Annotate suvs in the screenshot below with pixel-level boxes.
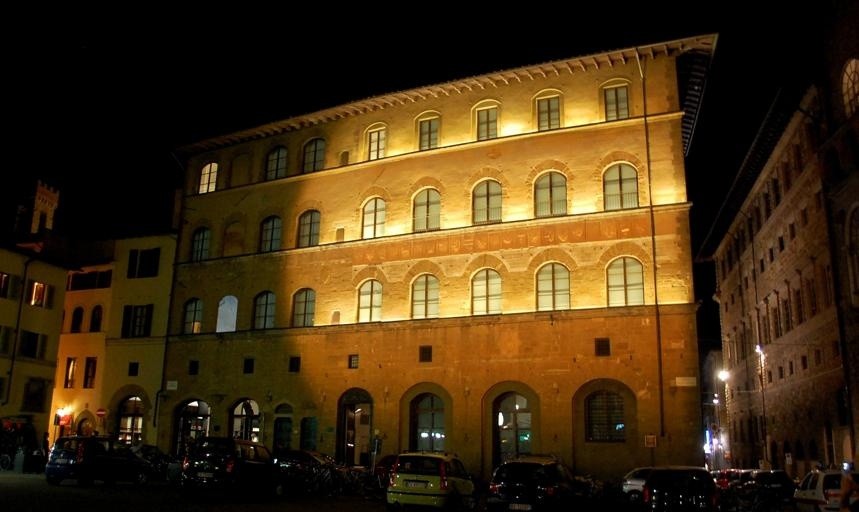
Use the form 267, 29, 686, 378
618, 465, 858, 512
386, 449, 605, 512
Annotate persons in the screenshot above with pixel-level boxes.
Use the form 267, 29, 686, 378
0, 415, 39, 474
39, 432, 51, 472
233, 431, 240, 440
91, 430, 98, 435
272, 439, 288, 458
250, 433, 258, 449
838, 459, 855, 512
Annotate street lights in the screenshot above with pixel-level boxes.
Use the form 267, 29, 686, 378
753, 344, 770, 462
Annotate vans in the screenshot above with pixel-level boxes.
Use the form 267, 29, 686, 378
179, 436, 271, 494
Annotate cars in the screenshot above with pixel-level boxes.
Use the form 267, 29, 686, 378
271, 448, 370, 499
45, 435, 180, 488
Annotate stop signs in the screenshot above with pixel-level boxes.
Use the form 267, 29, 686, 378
96, 407, 108, 419
